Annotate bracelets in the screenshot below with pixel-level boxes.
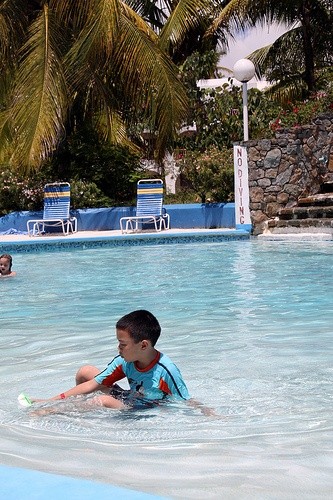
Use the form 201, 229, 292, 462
60, 393, 65, 400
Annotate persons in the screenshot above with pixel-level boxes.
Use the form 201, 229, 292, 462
0, 254, 17, 276
26, 309, 213, 420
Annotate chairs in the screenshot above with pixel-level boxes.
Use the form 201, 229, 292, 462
119, 179, 170, 234
27, 181, 78, 236
